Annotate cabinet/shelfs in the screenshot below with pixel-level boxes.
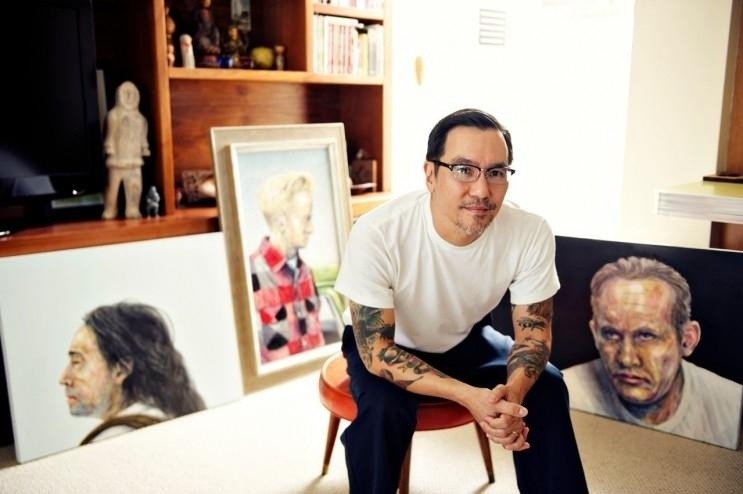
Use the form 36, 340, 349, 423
0, 0, 393, 258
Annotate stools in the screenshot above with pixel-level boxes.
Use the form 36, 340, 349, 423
318, 351, 504, 494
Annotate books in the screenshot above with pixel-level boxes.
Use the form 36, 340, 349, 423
313, 14, 384, 79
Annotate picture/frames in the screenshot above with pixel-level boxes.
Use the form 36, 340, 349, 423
206, 121, 365, 396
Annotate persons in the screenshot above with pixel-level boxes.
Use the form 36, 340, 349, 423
249, 176, 326, 365
60, 302, 207, 445
334, 108, 589, 494
146, 185, 160, 218
559, 256, 743, 450
102, 82, 151, 219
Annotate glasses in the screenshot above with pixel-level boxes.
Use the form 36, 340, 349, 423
433, 159, 516, 181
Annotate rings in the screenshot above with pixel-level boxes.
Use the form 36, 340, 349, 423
512, 430, 519, 438
